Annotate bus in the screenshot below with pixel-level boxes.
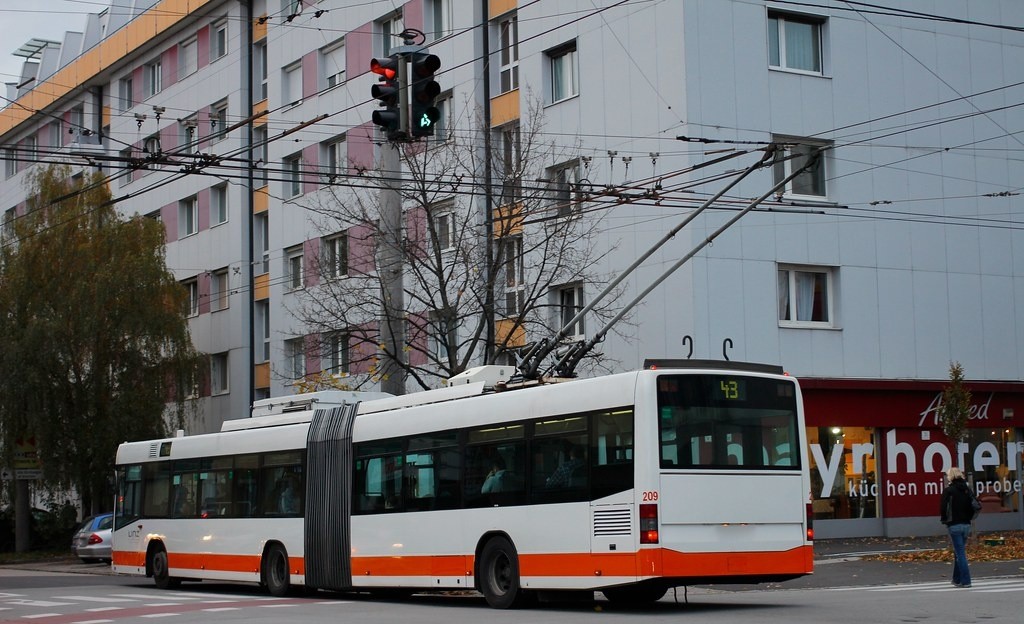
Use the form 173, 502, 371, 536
113, 337, 818, 605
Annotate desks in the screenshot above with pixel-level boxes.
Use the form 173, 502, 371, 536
979, 497, 1001, 512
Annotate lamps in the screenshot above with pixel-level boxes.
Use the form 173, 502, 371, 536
1002, 408, 1013, 420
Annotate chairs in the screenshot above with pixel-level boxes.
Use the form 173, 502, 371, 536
572, 465, 595, 486
502, 470, 526, 491
437, 480, 461, 497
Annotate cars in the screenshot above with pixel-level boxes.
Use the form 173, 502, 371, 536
70, 514, 117, 560
76, 514, 139, 566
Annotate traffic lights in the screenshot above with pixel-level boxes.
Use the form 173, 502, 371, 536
369, 54, 402, 139
410, 49, 441, 139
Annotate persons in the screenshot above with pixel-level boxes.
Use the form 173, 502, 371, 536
940, 467, 982, 588
280, 474, 300, 514
480, 456, 512, 494
545, 446, 586, 487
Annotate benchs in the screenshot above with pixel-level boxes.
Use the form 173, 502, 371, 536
1001, 507, 1011, 512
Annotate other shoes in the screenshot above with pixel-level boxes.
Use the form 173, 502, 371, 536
959, 584, 971, 588
951, 580, 960, 586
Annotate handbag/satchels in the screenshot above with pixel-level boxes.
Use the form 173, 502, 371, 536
970, 496, 981, 520
